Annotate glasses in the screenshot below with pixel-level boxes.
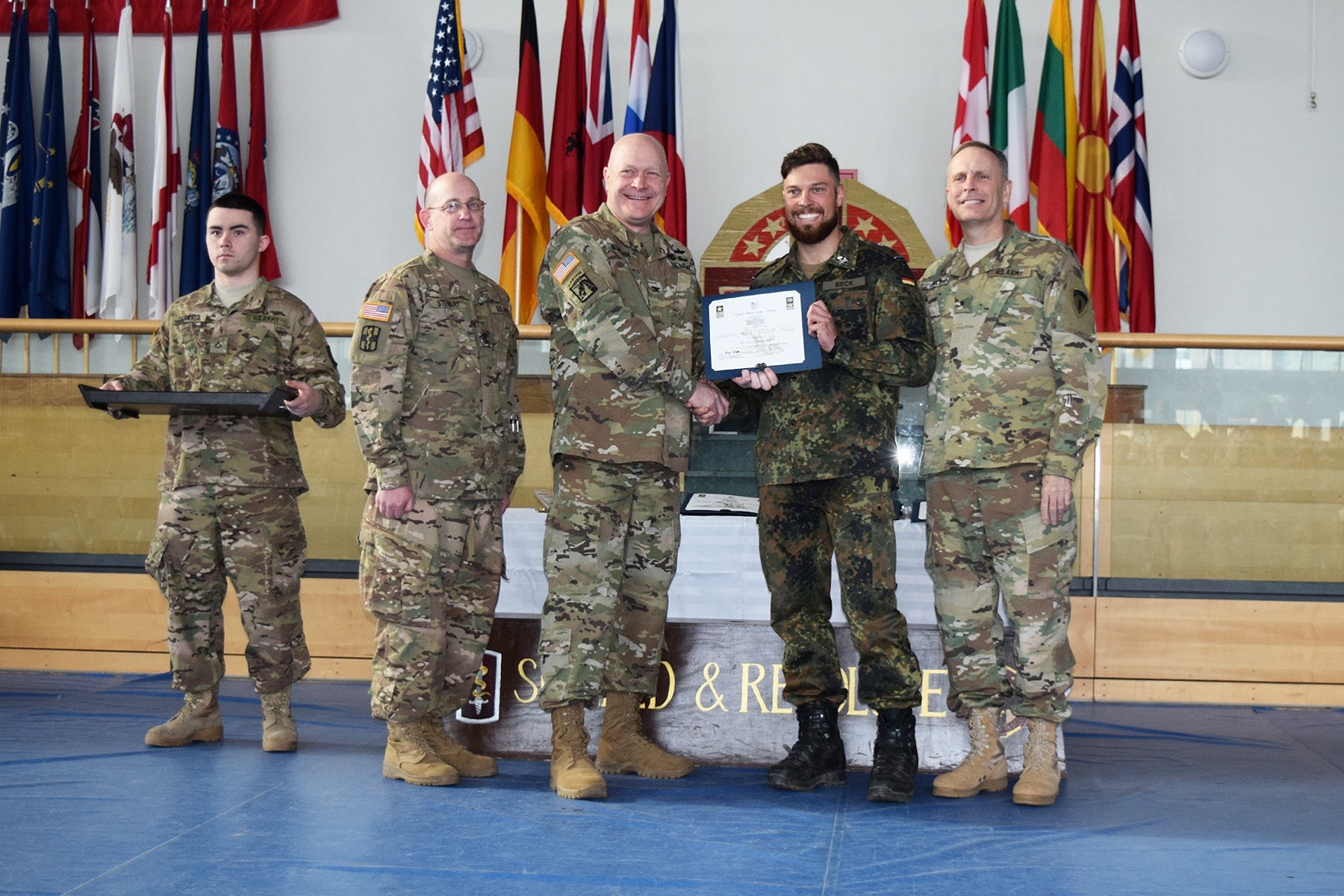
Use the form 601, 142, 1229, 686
429, 200, 486, 213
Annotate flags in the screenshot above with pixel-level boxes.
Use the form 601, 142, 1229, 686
1108, 0, 1156, 334
582, 0, 615, 217
991, 0, 1120, 353
944, 0, 996, 250
642, 0, 687, 245
546, 0, 588, 229
499, 0, 552, 323
623, 0, 651, 134
414, 0, 485, 249
0, 0, 281, 350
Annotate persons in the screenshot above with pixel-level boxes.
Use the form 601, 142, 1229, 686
96, 194, 347, 754
539, 133, 737, 800
684, 142, 937, 802
352, 172, 526, 783
915, 142, 1108, 802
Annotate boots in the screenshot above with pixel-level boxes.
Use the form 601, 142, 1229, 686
260, 688, 297, 751
932, 708, 1008, 797
769, 704, 846, 790
146, 689, 224, 747
866, 705, 918, 804
549, 702, 608, 800
383, 718, 460, 786
419, 717, 498, 777
1013, 715, 1059, 806
594, 690, 693, 780
